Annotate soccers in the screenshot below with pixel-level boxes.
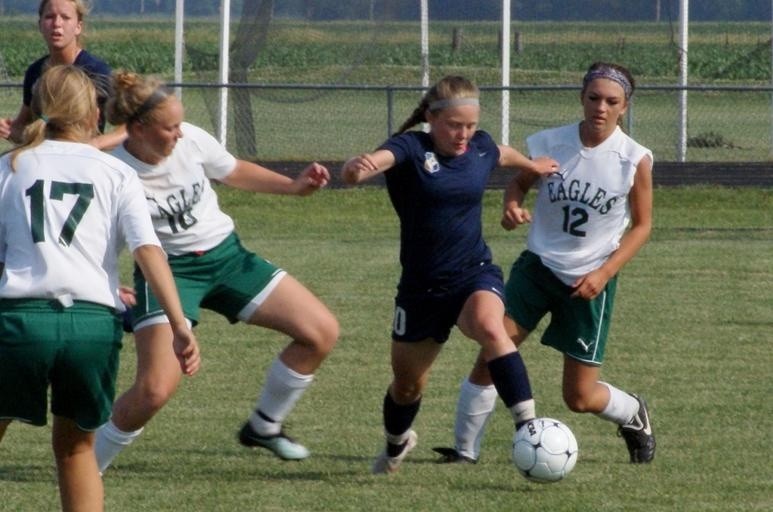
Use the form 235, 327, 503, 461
513, 417, 577, 484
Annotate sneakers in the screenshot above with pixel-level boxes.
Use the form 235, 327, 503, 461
433, 446, 475, 465
618, 393, 655, 464
374, 431, 416, 474
240, 421, 310, 461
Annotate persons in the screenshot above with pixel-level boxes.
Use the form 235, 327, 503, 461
431, 61, 655, 465
0, 0, 113, 144
95, 65, 340, 476
341, 75, 559, 478
0, 66, 201, 511
